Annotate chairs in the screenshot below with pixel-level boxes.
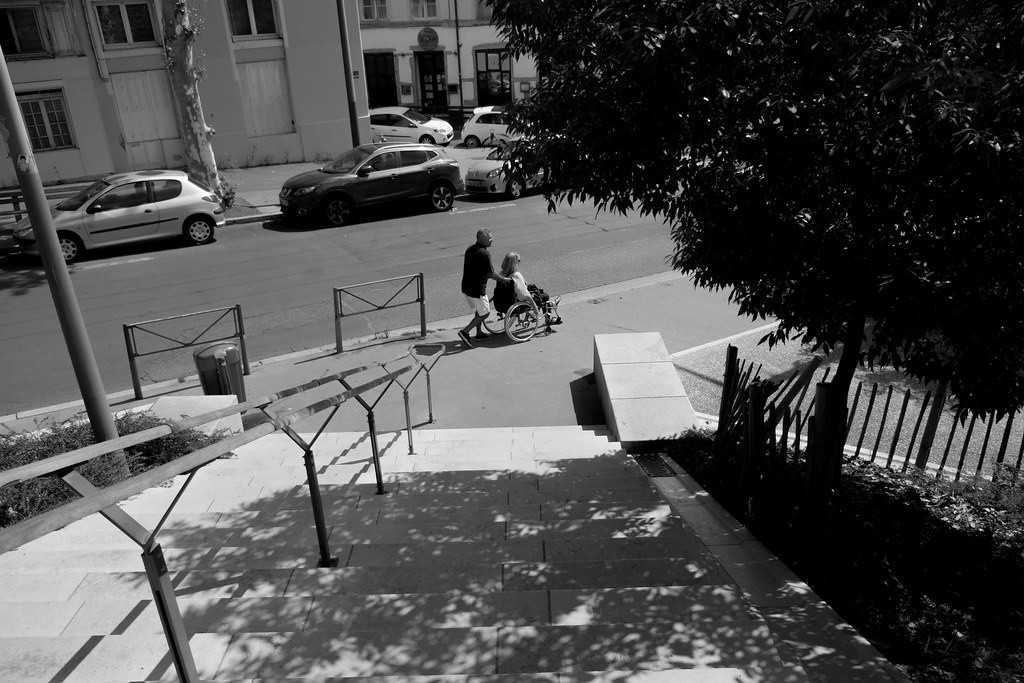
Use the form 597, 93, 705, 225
134, 182, 146, 202
386, 154, 394, 165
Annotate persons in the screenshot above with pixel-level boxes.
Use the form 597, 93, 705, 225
458, 227, 511, 348
498, 252, 561, 325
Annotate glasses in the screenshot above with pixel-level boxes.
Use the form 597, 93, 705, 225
517, 260, 520, 262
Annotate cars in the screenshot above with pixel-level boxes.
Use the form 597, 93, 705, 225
461, 106, 567, 149
13, 170, 226, 264
279, 142, 466, 226
466, 134, 588, 199
369, 106, 454, 144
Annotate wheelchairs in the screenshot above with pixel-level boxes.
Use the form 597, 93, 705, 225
483, 277, 563, 342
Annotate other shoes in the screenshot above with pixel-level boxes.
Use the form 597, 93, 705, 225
474, 332, 492, 341
458, 330, 473, 347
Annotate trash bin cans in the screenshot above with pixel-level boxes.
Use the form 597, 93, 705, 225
192, 340, 248, 415
